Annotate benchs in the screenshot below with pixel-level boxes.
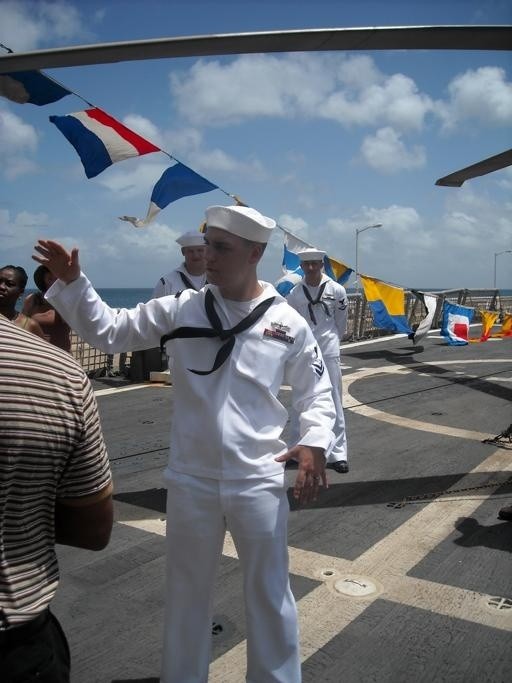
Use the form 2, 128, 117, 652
490, 313, 511, 338
48, 105, 160, 180
440, 300, 477, 348
323, 254, 353, 291
118, 163, 219, 229
0, 69, 74, 105
470, 308, 501, 342
360, 274, 416, 334
407, 290, 438, 345
274, 232, 312, 298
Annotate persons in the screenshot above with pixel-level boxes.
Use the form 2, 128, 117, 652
284, 249, 350, 476
31, 203, 339, 682
0, 312, 115, 682
21, 264, 71, 356
149, 229, 210, 301
0, 264, 43, 338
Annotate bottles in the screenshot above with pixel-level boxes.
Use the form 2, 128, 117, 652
208, 206, 276, 242
296, 248, 325, 260
175, 232, 207, 247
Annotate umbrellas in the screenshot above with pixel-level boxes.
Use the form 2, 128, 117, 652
328, 460, 350, 472
285, 459, 299, 469
